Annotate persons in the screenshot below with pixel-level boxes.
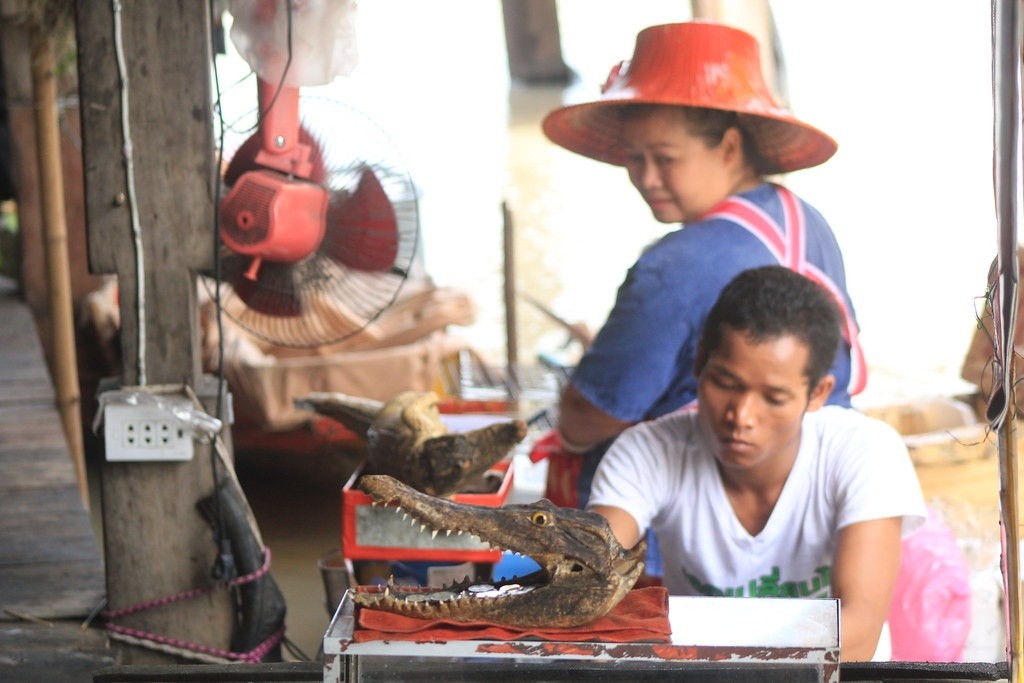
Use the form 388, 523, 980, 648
585, 267, 928, 663
542, 21, 866, 577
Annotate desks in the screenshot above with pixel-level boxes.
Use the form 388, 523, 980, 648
340, 414, 561, 592
322, 588, 842, 682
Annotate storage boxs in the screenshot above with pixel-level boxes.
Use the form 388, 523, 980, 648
92, 260, 445, 456
342, 456, 516, 564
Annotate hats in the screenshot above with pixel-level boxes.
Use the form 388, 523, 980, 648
540, 22, 837, 174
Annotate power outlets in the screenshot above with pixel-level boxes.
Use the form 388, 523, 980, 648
104, 402, 195, 460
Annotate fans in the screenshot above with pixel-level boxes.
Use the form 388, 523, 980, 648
200, 0, 420, 347
